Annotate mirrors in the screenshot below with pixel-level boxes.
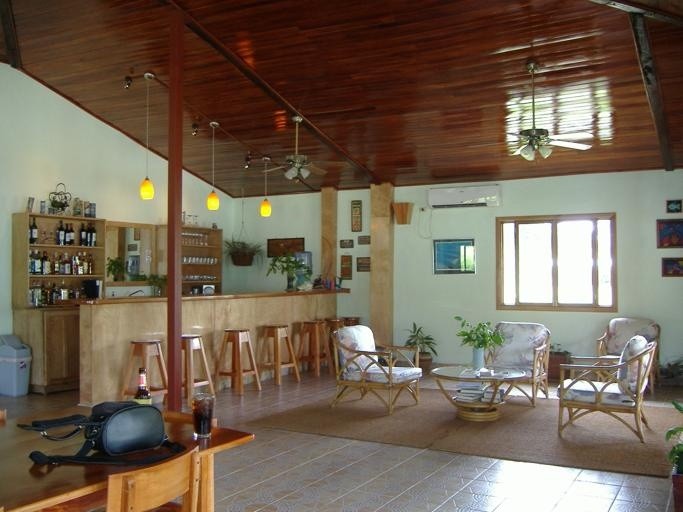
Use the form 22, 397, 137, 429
101, 220, 157, 286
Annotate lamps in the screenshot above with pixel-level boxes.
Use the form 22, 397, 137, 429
283, 166, 310, 181
519, 139, 553, 161
137, 72, 271, 220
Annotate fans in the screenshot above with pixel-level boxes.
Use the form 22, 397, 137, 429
504, 61, 592, 155
255, 115, 328, 183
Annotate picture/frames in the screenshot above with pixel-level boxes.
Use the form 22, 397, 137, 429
663, 197, 683, 215
431, 238, 476, 276
266, 237, 304, 259
659, 257, 683, 278
127, 228, 141, 275
336, 199, 369, 280
656, 218, 683, 248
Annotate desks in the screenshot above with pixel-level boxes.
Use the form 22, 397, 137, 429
0, 404, 255, 512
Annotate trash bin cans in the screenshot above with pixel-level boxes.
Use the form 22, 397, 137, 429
0, 334, 32, 396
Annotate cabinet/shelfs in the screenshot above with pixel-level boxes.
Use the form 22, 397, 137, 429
11, 212, 103, 308
548, 348, 569, 381
154, 223, 222, 294
10, 307, 79, 397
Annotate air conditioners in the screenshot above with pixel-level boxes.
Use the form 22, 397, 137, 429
427, 185, 500, 209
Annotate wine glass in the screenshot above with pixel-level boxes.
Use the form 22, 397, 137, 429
186, 214, 200, 228
181, 232, 210, 247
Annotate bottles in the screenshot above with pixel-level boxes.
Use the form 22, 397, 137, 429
28, 250, 95, 276
30, 218, 38, 245
28, 279, 87, 308
320, 274, 335, 289
133, 366, 153, 405
56, 217, 96, 247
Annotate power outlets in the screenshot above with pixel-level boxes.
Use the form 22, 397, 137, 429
419, 207, 426, 214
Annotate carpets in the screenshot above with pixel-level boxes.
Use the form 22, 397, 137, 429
246, 386, 682, 480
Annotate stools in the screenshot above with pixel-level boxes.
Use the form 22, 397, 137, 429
120, 317, 362, 406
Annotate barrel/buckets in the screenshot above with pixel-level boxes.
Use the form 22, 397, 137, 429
295, 252, 312, 280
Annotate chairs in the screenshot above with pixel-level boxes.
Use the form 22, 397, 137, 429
103, 446, 200, 512
474, 323, 552, 407
593, 317, 661, 391
560, 334, 658, 440
328, 324, 421, 411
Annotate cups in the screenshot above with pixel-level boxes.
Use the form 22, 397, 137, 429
181, 255, 220, 265
190, 391, 214, 440
151, 287, 161, 296
181, 211, 185, 225
183, 273, 216, 282
192, 287, 200, 294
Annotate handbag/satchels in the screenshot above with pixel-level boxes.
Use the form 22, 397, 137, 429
83, 400, 166, 458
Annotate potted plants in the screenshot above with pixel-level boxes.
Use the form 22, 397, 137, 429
266, 254, 309, 292
104, 256, 127, 282
404, 321, 437, 370
451, 316, 505, 371
663, 398, 682, 512
224, 239, 263, 266
